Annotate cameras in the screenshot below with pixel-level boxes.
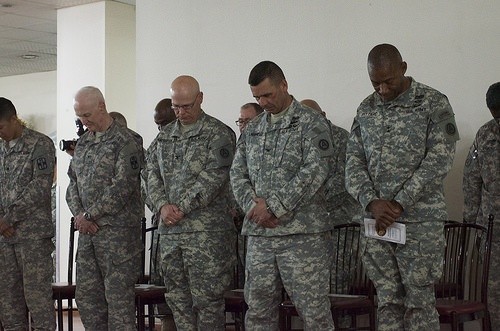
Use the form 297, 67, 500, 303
59, 118, 86, 151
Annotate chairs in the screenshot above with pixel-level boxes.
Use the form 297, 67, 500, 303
222, 215, 247, 331
134, 218, 178, 331
52, 217, 76, 331
329, 221, 378, 331
433, 214, 491, 331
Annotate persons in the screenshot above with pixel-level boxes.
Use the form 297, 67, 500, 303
67, 112, 143, 156
298, 99, 363, 328
231, 104, 264, 331
464, 81, 500, 331
230, 61, 335, 331
145, 98, 176, 331
140, 75, 236, 331
65, 86, 143, 331
0, 97, 56, 331
346, 43, 461, 331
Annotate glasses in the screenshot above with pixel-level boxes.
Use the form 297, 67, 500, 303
171, 92, 200, 110
235, 118, 252, 126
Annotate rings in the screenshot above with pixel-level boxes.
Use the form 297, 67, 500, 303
169, 221, 172, 225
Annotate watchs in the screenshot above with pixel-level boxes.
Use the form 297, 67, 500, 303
83, 212, 91, 220
267, 206, 273, 215
